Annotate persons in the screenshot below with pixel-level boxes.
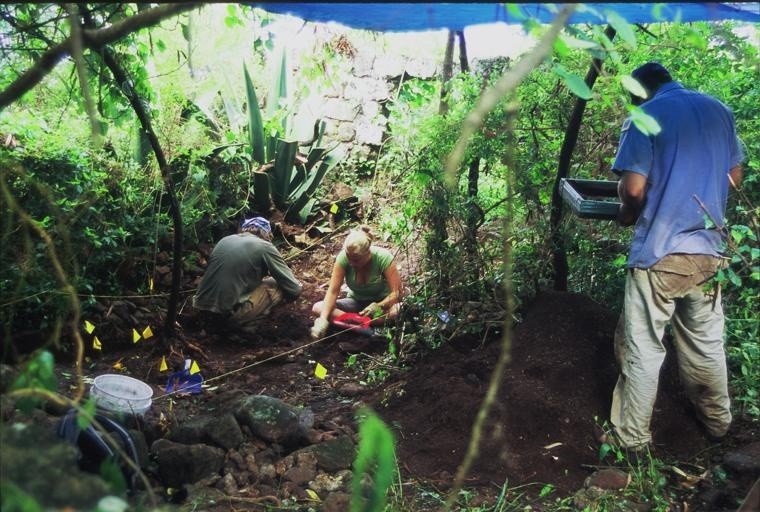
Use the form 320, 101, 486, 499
311, 225, 410, 339
592, 61, 743, 453
193, 216, 302, 341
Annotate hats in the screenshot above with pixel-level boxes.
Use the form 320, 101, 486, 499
241, 215, 271, 233
630, 62, 669, 105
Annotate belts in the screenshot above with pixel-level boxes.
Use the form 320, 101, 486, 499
201, 304, 240, 322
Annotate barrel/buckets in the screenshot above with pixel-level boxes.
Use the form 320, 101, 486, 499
90, 374, 154, 420
